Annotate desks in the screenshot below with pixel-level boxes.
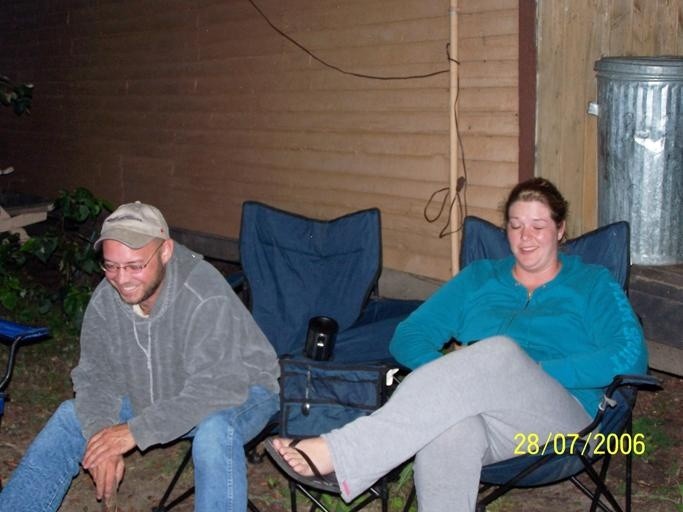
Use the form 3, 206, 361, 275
282, 316, 408, 511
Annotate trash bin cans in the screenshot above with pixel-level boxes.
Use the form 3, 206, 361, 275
594, 56, 683, 267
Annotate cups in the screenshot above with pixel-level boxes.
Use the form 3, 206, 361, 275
304, 316, 339, 361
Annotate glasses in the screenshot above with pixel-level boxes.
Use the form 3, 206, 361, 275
100, 242, 162, 273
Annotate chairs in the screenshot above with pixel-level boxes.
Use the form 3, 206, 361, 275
158, 202, 380, 512
0, 317, 51, 417
404, 217, 662, 511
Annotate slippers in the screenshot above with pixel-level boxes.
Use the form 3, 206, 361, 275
264, 436, 341, 493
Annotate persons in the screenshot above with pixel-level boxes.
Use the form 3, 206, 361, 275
0, 200, 282, 511
263, 177, 648, 512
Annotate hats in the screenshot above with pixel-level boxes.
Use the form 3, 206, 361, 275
94, 201, 170, 248
